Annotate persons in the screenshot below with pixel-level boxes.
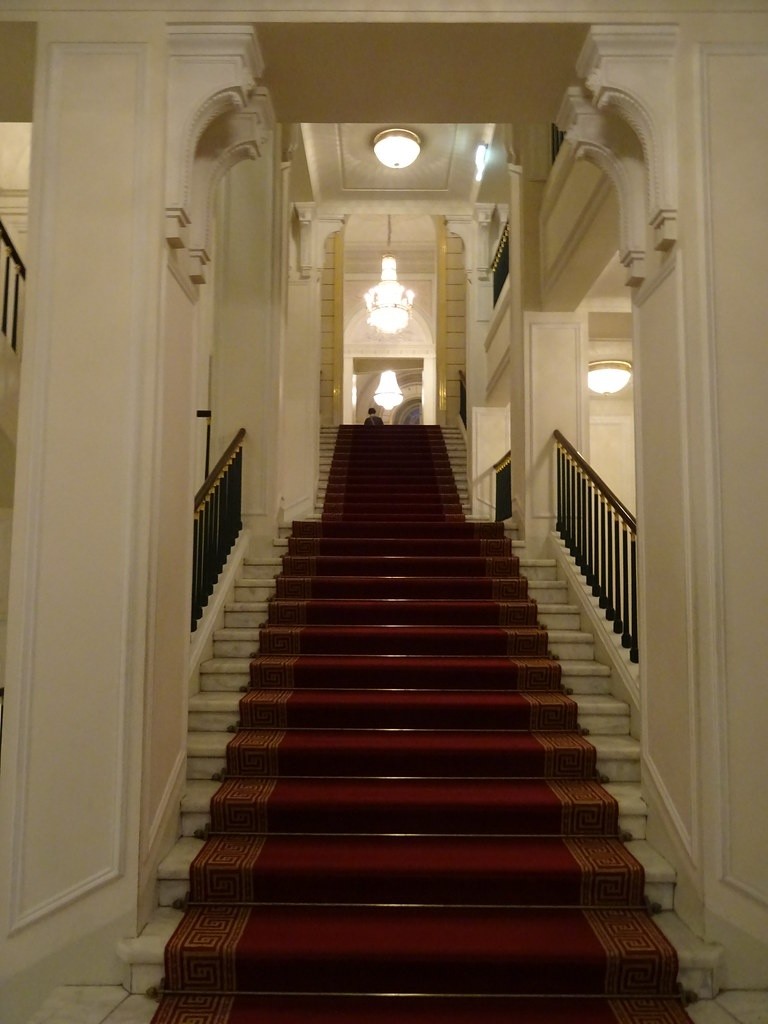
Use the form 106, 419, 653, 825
363, 408, 384, 425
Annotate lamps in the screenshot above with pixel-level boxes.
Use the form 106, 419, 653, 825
364, 253, 414, 335
588, 361, 632, 396
374, 369, 404, 410
373, 128, 421, 168
